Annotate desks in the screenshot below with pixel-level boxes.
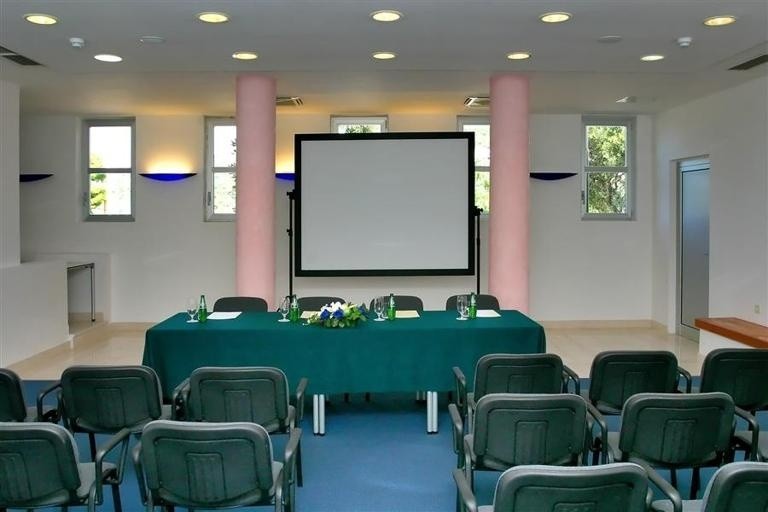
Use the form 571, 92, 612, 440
67, 262, 96, 321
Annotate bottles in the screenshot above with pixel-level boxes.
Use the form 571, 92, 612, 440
387, 293, 396, 320
199, 295, 207, 322
290, 294, 299, 320
468, 292, 477, 318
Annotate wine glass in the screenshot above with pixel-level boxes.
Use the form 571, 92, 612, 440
456, 296, 468, 321
278, 297, 290, 322
186, 297, 199, 323
372, 298, 386, 321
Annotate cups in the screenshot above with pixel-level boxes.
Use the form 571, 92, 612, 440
382, 302, 388, 319
462, 301, 470, 317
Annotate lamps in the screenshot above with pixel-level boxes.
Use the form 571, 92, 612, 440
20, 174, 53, 182
275, 172, 297, 181
529, 172, 578, 180
139, 173, 197, 182
